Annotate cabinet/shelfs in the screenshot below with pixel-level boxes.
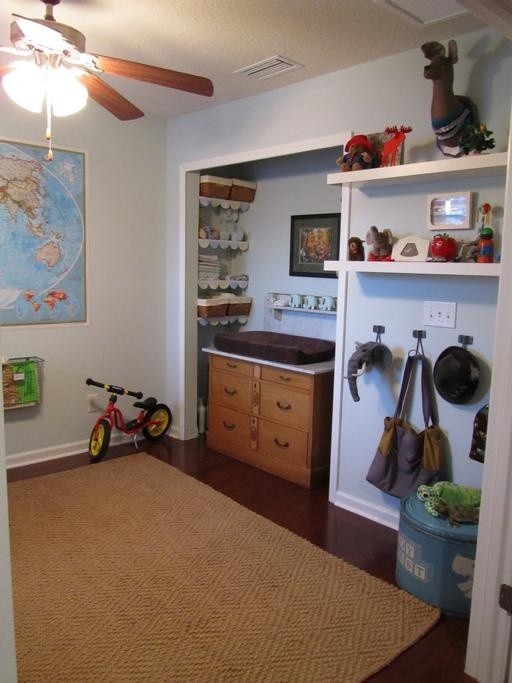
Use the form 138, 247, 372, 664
197, 196, 251, 325
201, 347, 334, 490
323, 151, 509, 530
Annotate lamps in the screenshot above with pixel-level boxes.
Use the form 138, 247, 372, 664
2, 48, 89, 161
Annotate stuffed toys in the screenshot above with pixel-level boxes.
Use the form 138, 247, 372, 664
348, 237, 364, 261
343, 340, 393, 402
364, 225, 394, 262
334, 134, 374, 172
417, 480, 481, 520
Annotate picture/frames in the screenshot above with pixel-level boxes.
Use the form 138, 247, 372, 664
289, 213, 340, 278
0, 136, 90, 329
427, 192, 472, 231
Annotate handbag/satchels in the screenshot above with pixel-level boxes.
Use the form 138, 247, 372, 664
365, 355, 445, 500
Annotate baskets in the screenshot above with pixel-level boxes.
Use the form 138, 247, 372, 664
229, 177, 257, 201
3, 356, 45, 411
209, 289, 252, 315
197, 293, 230, 317
200, 174, 233, 199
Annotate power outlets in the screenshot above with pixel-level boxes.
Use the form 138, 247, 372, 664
423, 301, 457, 328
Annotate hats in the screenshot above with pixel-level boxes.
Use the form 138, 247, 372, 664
433, 346, 479, 405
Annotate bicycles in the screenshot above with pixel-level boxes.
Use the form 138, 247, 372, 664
86, 378, 172, 463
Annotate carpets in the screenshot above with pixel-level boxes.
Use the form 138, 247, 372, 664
7, 452, 440, 683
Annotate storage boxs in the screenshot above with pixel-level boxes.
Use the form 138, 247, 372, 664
395, 494, 477, 618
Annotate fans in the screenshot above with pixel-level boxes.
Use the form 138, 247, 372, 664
0, 0, 213, 120
